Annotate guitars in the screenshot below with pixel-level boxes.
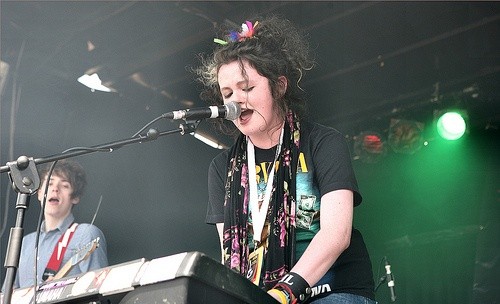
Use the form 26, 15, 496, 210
54, 237, 100, 279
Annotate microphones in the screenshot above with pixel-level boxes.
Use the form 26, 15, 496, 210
161, 101, 241, 122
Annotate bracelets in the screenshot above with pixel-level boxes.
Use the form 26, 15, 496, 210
267, 272, 312, 304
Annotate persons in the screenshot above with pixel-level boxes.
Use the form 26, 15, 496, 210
11, 158, 107, 288
186, 14, 378, 304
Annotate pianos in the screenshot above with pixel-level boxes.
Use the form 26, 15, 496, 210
1, 251, 283, 304
